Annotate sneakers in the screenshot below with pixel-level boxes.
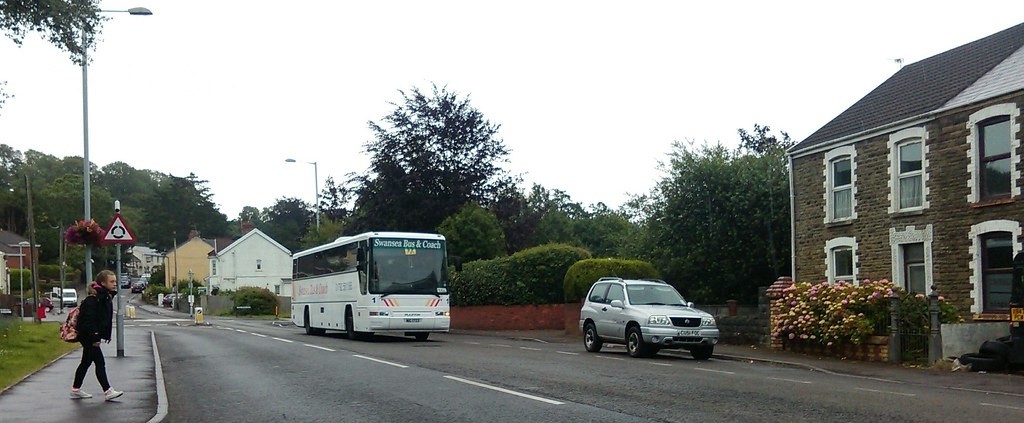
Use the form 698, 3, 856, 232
70, 387, 92, 398
104, 387, 124, 401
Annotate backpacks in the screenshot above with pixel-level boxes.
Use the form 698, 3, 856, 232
60, 293, 97, 343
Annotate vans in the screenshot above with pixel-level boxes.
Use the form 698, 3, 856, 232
44, 292, 62, 302
61, 288, 79, 308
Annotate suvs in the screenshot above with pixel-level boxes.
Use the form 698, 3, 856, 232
579, 276, 720, 360
16, 297, 54, 313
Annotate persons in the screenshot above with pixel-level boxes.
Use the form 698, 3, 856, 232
69, 270, 122, 401
38, 302, 47, 324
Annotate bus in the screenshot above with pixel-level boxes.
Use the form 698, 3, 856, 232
290, 231, 463, 341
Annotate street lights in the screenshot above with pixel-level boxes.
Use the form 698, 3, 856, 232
82, 6, 153, 299
285, 158, 320, 233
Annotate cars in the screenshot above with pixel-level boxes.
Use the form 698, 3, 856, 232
120, 273, 151, 293
163, 293, 182, 305
